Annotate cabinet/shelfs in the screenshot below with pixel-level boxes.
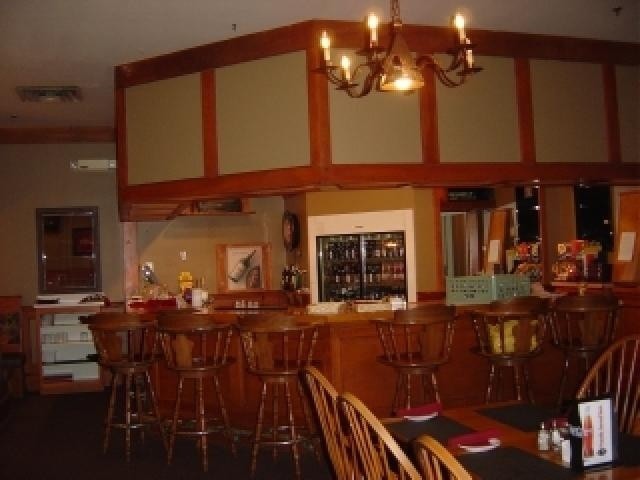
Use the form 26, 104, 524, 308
316, 232, 406, 303
17, 302, 105, 398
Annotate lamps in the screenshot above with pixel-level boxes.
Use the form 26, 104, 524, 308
305, 0, 487, 100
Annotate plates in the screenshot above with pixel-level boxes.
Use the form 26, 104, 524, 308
405, 412, 438, 422
459, 438, 499, 453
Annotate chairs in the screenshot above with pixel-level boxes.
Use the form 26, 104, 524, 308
97, 293, 639, 479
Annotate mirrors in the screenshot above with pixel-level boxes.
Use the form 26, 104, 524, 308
35, 205, 102, 294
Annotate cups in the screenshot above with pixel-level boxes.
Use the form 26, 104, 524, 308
80, 332, 90, 341
191, 288, 204, 309
43, 331, 68, 343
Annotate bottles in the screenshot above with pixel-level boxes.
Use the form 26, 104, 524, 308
281, 264, 303, 291
199, 277, 209, 305
231, 249, 257, 282
537, 418, 582, 471
